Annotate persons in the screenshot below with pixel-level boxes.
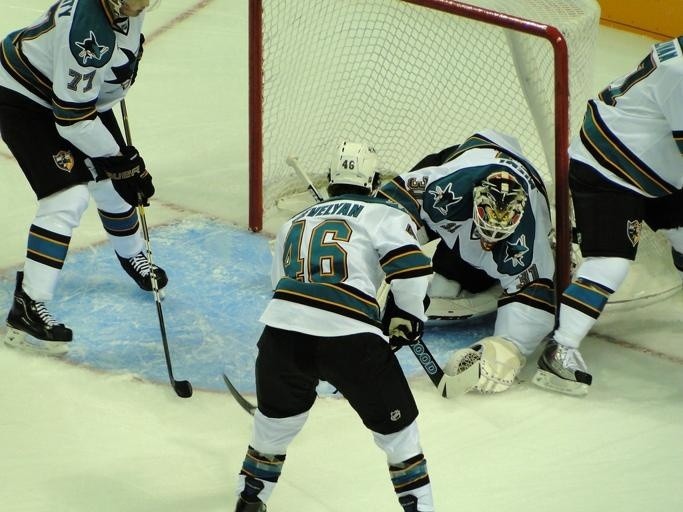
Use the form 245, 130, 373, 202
0, 0, 169, 343
377, 130, 584, 393
234, 141, 435, 512
536, 36, 683, 386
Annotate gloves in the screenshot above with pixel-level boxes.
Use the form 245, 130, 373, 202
103, 147, 155, 207
467, 336, 526, 394
384, 310, 424, 347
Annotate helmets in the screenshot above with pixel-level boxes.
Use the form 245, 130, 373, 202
472, 171, 527, 244
327, 140, 383, 199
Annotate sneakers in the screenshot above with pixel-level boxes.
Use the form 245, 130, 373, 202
115, 250, 167, 291
537, 338, 592, 385
6, 272, 73, 342
